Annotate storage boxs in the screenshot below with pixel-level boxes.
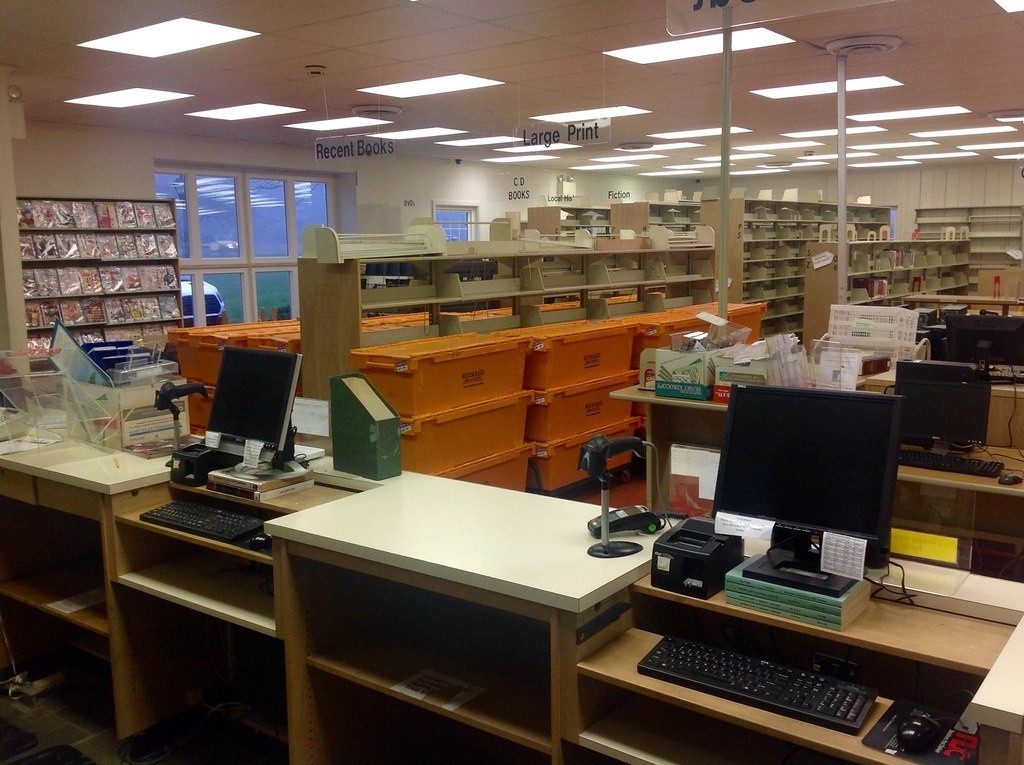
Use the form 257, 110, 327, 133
826, 304, 919, 346
168, 301, 764, 495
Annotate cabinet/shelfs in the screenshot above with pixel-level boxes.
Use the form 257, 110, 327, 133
523, 180, 974, 345
13, 195, 186, 358
298, 220, 725, 459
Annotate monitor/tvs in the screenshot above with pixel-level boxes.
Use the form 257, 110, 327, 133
710, 316, 1024, 597
208, 346, 303, 451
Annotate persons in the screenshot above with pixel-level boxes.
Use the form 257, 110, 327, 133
163, 268, 176, 289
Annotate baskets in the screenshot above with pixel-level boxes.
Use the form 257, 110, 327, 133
827, 303, 919, 381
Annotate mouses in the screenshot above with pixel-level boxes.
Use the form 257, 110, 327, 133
998, 473, 1023, 485
249, 531, 272, 551
896, 712, 945, 756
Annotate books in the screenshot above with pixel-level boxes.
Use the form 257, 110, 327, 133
913, 276, 922, 291
17, 201, 180, 357
670, 331, 709, 352
854, 276, 887, 299
725, 553, 872, 632
883, 248, 915, 269
206, 467, 315, 501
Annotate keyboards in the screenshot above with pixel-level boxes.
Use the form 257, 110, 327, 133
139, 500, 264, 544
897, 449, 1005, 478
988, 372, 1024, 384
638, 633, 880, 733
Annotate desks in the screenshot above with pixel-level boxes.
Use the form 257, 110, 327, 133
899, 295, 1024, 317
885, 444, 1024, 557
609, 372, 868, 518
868, 373, 1024, 448
575, 562, 1024, 765
0, 422, 683, 764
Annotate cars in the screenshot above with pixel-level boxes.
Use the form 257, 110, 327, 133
179, 274, 226, 328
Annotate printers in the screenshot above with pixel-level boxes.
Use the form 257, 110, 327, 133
650, 516, 746, 600
